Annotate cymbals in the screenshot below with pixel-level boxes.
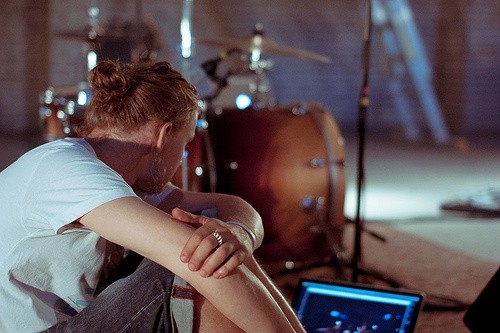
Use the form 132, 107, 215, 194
47, 23, 127, 46
195, 30, 333, 71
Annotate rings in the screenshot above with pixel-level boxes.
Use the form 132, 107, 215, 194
212, 231, 223, 245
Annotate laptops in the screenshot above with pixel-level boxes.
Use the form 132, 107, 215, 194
292, 277, 423, 333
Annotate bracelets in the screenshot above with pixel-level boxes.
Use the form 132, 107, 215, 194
226, 221, 258, 252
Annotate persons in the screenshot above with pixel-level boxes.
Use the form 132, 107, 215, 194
0, 59, 308, 333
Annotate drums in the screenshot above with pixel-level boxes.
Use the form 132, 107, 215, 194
188, 102, 347, 290
45, 76, 213, 184
